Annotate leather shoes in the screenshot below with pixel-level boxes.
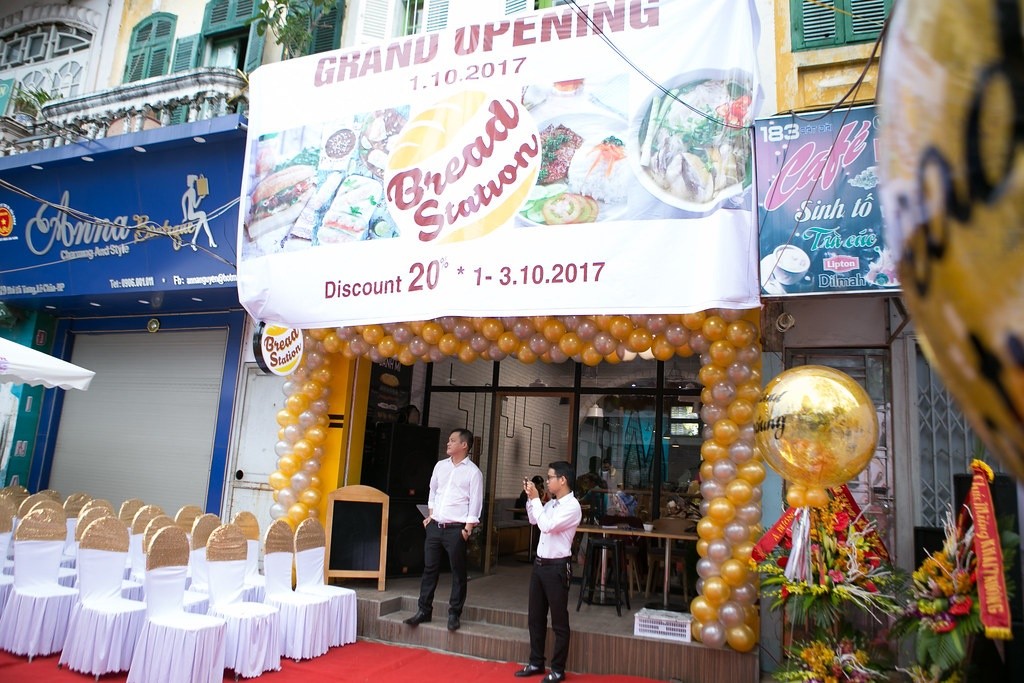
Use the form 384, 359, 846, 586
541, 670, 565, 683
447, 615, 460, 631
402, 608, 432, 625
514, 663, 545, 676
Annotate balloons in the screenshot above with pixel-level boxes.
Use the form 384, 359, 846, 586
691, 306, 762, 652
753, 363, 879, 489
331, 313, 691, 365
269, 327, 330, 532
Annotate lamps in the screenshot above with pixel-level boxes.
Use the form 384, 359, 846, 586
530, 359, 548, 387
585, 365, 603, 417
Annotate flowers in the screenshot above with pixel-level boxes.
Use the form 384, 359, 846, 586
747, 484, 1020, 683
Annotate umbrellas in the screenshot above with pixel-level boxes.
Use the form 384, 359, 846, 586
0, 335, 97, 393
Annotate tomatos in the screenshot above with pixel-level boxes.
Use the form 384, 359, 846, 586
542, 193, 598, 224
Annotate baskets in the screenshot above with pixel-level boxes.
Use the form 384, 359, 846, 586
632, 607, 695, 643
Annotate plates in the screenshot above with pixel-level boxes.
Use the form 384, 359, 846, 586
760, 253, 814, 295
514, 111, 656, 226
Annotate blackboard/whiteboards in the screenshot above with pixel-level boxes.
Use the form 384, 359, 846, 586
324, 484, 390, 578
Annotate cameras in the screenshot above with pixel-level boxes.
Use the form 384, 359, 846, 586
525, 479, 528, 486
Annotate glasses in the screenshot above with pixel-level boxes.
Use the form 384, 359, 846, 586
547, 474, 567, 480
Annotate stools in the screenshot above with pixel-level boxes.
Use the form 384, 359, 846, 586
577, 538, 632, 618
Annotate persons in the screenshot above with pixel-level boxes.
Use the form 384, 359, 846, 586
518, 475, 558, 556
514, 460, 583, 683
402, 427, 484, 631
568, 456, 609, 591
599, 457, 623, 490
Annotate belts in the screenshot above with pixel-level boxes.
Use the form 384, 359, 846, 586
430, 518, 466, 529
536, 556, 571, 565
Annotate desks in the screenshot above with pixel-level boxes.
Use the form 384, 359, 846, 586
573, 524, 700, 611
505, 507, 534, 565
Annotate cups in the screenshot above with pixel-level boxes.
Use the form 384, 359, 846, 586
772, 244, 814, 287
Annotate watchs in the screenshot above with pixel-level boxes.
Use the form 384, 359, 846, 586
464, 528, 472, 536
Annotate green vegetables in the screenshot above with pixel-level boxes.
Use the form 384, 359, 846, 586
536, 133, 624, 180
343, 179, 377, 216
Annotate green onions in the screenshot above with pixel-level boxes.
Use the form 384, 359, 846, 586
657, 107, 744, 172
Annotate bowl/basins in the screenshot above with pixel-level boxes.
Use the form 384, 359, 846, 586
625, 62, 760, 214
643, 524, 654, 532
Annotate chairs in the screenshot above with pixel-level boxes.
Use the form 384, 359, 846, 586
635, 518, 697, 603
0, 485, 359, 683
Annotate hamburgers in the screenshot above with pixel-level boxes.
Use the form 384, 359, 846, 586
245, 165, 316, 239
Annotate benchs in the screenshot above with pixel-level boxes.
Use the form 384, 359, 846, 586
493, 519, 531, 555
